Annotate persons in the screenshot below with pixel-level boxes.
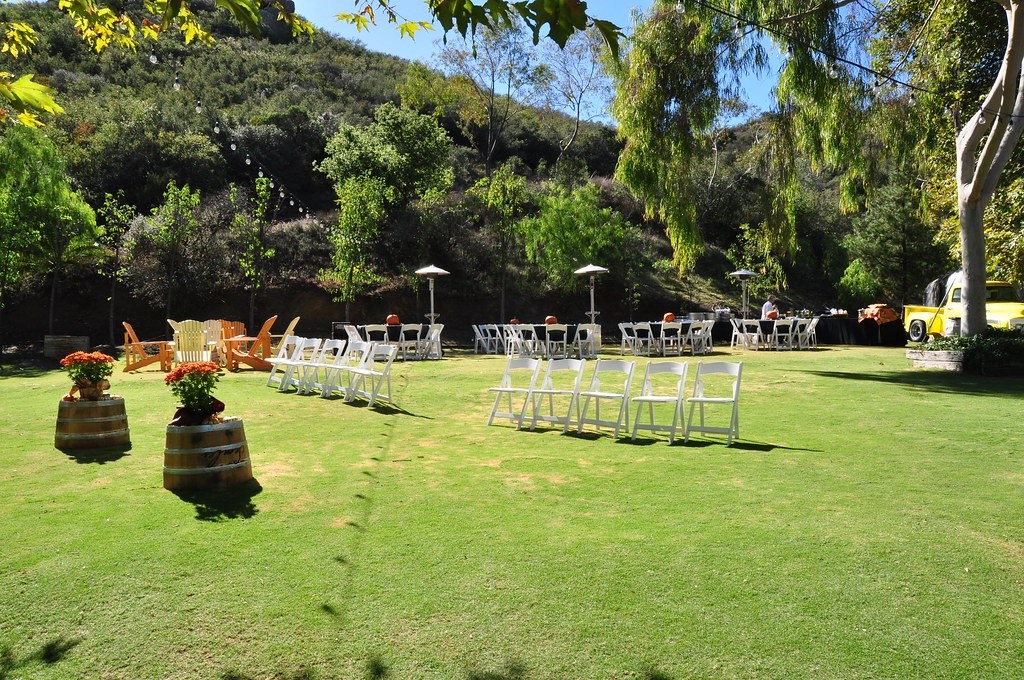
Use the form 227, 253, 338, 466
760, 294, 779, 346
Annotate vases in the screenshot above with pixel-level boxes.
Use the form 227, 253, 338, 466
54, 396, 131, 450
162, 418, 253, 491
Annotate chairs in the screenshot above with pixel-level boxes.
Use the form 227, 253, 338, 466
303, 339, 347, 396
344, 326, 365, 361
270, 316, 301, 358
471, 323, 596, 358
365, 324, 391, 361
420, 324, 444, 360
619, 318, 715, 356
396, 323, 423, 361
577, 359, 635, 438
122, 322, 169, 370
218, 319, 233, 369
231, 315, 278, 371
169, 319, 217, 370
348, 343, 398, 407
631, 361, 687, 445
231, 322, 247, 350
266, 334, 308, 390
684, 361, 743, 446
529, 359, 587, 433
487, 358, 542, 429
729, 318, 819, 351
203, 320, 224, 361
324, 339, 375, 401
281, 339, 323, 394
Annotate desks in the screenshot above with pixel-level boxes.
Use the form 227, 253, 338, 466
168, 340, 217, 365
516, 325, 588, 359
481, 325, 517, 353
357, 325, 430, 359
625, 321, 710, 353
745, 316, 906, 347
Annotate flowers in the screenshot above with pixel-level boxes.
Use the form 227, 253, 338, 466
57, 350, 115, 398
164, 360, 224, 422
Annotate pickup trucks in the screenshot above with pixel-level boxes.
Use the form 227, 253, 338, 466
904, 281, 1023, 342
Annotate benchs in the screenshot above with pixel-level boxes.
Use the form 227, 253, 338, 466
40, 336, 89, 370
